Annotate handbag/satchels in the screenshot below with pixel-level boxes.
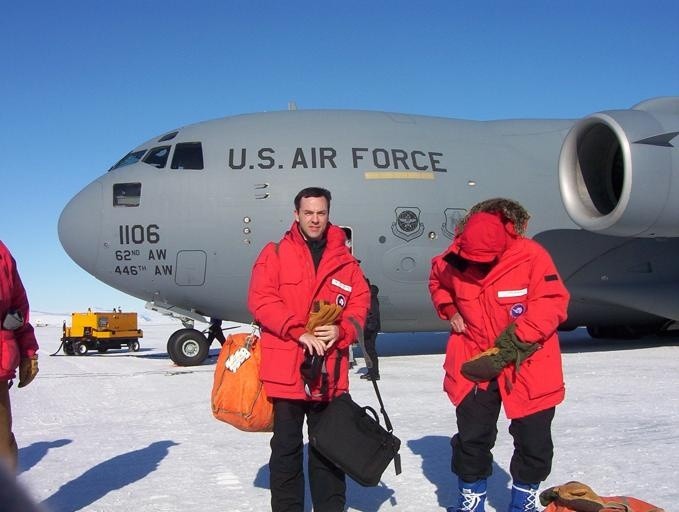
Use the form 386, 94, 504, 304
211, 319, 276, 432
309, 317, 402, 487
545, 494, 654, 512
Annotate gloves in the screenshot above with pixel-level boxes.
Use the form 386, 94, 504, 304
461, 322, 543, 393
539, 481, 604, 511
303, 299, 343, 369
18, 354, 40, 388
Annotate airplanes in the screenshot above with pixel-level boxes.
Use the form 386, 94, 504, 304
54, 86, 679, 370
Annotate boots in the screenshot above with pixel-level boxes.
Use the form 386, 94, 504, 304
456, 476, 487, 512
508, 479, 540, 512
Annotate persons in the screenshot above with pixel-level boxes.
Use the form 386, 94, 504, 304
246, 186, 371, 512
357, 277, 381, 380
0, 240, 39, 481
428, 198, 570, 511
208, 318, 227, 347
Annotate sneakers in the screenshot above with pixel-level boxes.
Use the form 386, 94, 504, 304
360, 372, 371, 379
367, 374, 380, 380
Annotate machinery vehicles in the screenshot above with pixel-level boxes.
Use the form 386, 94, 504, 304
58, 306, 143, 356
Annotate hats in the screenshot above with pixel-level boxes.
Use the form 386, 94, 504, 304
458, 212, 507, 264
